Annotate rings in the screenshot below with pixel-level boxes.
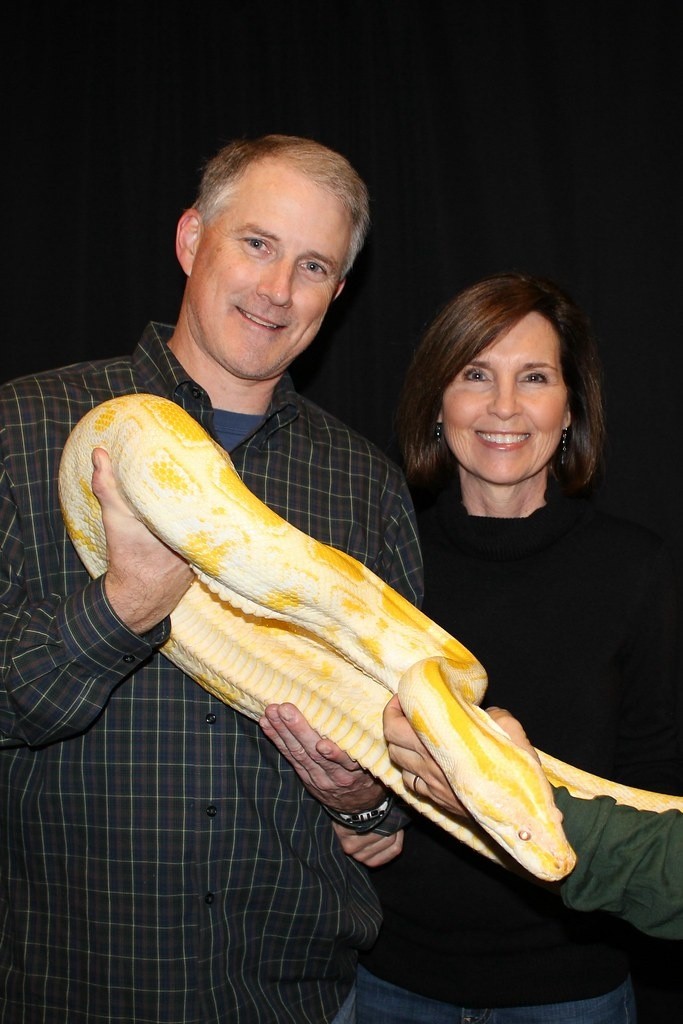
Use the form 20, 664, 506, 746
413, 776, 419, 791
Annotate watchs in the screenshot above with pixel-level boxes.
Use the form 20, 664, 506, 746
331, 794, 390, 823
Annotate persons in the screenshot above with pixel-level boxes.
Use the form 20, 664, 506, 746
0, 136, 683, 1023
